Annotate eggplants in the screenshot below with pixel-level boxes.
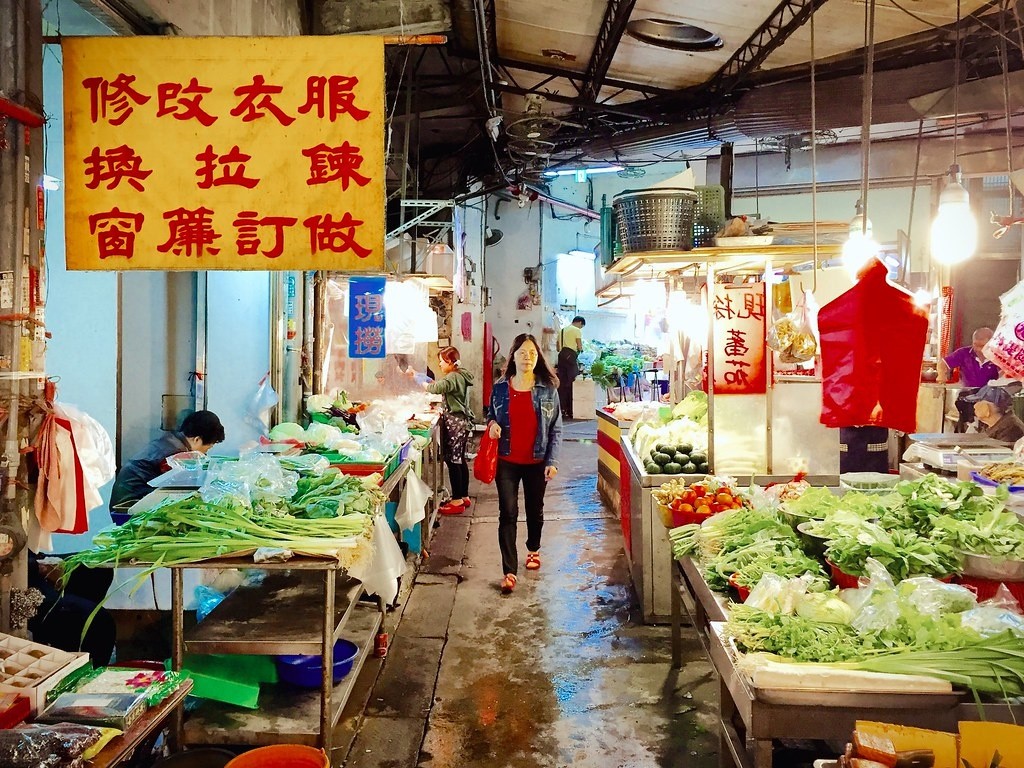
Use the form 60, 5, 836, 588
323, 405, 360, 430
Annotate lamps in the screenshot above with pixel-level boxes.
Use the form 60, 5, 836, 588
839, 0, 880, 270
925, 0, 978, 263
544, 165, 625, 182
569, 233, 595, 261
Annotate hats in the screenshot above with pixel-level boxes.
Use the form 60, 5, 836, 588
963, 385, 1012, 408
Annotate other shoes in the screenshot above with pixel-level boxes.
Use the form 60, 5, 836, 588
562, 411, 573, 418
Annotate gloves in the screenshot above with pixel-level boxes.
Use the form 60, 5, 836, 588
414, 372, 433, 386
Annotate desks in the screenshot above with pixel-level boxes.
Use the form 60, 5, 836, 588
620, 434, 711, 624
20, 667, 193, 768
572, 368, 671, 418
668, 461, 1024, 768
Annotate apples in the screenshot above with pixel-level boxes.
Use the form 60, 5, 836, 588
670, 485, 743, 513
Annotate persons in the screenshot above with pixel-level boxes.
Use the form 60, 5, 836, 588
396, 346, 475, 515
109, 410, 225, 514
936, 327, 1002, 388
375, 370, 384, 384
965, 385, 1024, 451
487, 333, 563, 591
556, 316, 585, 419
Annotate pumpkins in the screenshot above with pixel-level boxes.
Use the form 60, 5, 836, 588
642, 442, 708, 474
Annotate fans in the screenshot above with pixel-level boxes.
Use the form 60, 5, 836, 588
505, 93, 561, 158
617, 166, 646, 179
484, 225, 504, 247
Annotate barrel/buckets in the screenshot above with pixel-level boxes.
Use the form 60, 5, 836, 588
224, 743, 330, 768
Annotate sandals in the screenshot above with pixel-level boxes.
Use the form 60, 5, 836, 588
500, 574, 515, 592
526, 552, 541, 570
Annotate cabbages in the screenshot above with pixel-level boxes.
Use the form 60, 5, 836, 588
268, 422, 382, 462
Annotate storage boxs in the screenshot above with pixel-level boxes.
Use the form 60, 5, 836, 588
613, 185, 725, 257
573, 379, 608, 419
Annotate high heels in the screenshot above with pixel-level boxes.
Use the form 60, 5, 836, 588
464, 498, 471, 507
438, 502, 466, 514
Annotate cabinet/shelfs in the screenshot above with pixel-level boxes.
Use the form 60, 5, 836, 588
84, 403, 446, 768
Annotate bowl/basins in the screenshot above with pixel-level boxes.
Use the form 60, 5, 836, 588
651, 471, 1024, 617
272, 637, 359, 687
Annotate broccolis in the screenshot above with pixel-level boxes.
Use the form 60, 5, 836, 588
329, 417, 346, 429
346, 424, 358, 434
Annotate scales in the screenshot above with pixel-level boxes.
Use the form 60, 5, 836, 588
127, 470, 208, 516
902, 433, 1013, 476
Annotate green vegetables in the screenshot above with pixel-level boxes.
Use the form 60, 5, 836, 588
672, 389, 707, 425
592, 354, 644, 390
698, 473, 1024, 725
669, 507, 773, 560
50, 445, 375, 653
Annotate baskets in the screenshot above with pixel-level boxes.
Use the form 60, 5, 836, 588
612, 188, 699, 252
692, 185, 726, 249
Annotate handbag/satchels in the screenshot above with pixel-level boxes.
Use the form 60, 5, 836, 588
474, 421, 498, 483
558, 347, 578, 365
